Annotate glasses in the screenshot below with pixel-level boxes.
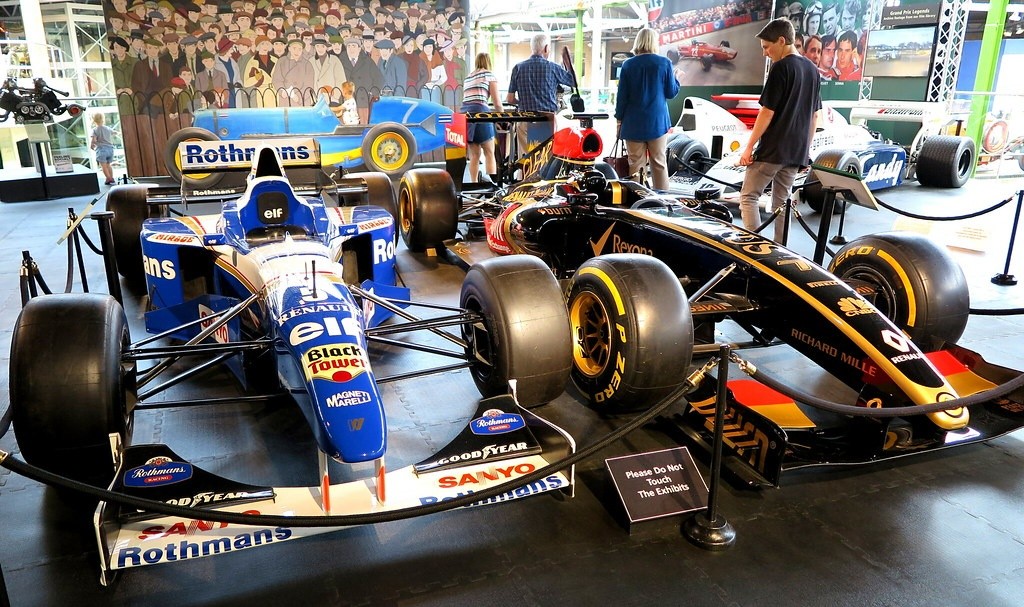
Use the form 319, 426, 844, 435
807, 2, 823, 14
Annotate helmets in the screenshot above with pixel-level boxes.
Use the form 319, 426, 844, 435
788, 2, 804, 19
803, 2, 822, 33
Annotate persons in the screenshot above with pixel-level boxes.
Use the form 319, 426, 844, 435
653, 0, 773, 46
614, 28, 685, 191
775, 0, 877, 81
91, 113, 119, 185
459, 53, 505, 198
507, 34, 578, 179
740, 18, 823, 243
329, 82, 360, 125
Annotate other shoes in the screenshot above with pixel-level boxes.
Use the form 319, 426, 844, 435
104, 177, 114, 185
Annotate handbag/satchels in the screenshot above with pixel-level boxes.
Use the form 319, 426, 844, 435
603, 137, 629, 178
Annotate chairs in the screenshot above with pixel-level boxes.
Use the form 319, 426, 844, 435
248, 144, 287, 181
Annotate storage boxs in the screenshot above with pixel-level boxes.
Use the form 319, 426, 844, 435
53, 154, 73, 173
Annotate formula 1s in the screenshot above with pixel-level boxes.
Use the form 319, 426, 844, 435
0, 93, 1024, 592
666, 40, 738, 73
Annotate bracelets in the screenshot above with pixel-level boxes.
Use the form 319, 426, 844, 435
617, 122, 621, 126
